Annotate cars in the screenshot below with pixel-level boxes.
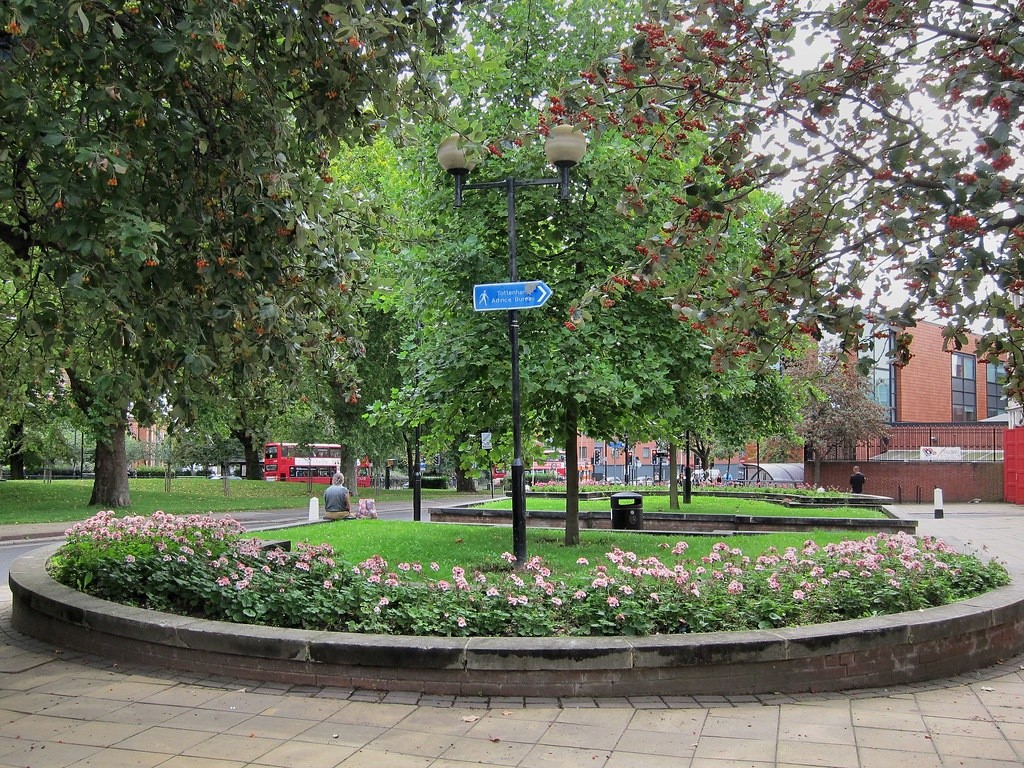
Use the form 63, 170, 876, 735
633, 476, 653, 486
599, 477, 622, 485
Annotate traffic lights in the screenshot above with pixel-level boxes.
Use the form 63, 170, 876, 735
594, 449, 600, 466
651, 450, 658, 465
629, 452, 633, 466
433, 454, 440, 466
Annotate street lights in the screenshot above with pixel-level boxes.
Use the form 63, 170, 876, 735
437, 124, 587, 559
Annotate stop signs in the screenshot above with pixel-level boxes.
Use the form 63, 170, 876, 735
740, 457, 745, 464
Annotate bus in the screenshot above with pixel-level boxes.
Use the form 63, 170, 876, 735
494, 461, 565, 487
264, 443, 370, 488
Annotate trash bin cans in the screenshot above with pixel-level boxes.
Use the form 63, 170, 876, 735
609, 492, 643, 531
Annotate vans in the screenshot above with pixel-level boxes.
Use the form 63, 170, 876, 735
691, 470, 720, 481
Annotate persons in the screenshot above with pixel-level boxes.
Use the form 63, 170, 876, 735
850, 466, 866, 493
324, 472, 351, 519
676, 469, 734, 487
